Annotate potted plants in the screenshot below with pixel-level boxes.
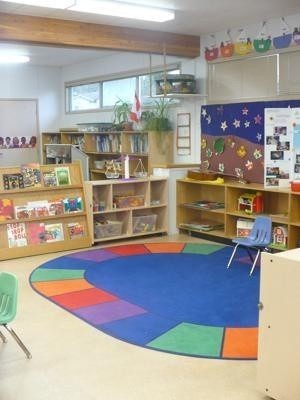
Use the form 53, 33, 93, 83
112, 96, 174, 131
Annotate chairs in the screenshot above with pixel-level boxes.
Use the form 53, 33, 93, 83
0, 270, 32, 359
227, 216, 273, 276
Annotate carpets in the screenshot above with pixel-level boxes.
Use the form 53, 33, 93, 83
28, 241, 260, 361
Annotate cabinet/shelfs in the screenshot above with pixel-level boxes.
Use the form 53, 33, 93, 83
0, 130, 300, 260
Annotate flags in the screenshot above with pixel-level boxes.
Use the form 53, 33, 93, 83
129, 93, 141, 123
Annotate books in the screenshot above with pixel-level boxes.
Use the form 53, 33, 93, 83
0, 134, 150, 248
177, 199, 225, 232
